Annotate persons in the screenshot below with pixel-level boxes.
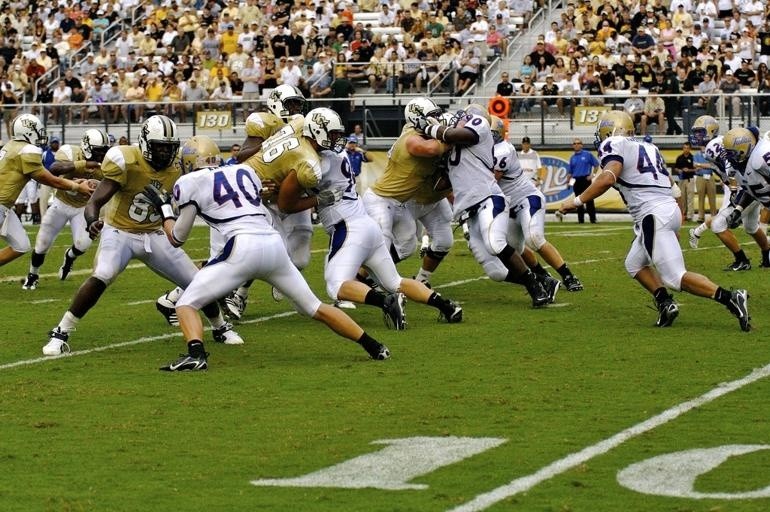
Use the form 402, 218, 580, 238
567, 138, 601, 224
42, 115, 245, 356
675, 115, 769, 271
1, 114, 129, 291
554, 107, 751, 331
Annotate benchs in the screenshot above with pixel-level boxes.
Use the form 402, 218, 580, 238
504, 0, 770, 120
0, 0, 550, 124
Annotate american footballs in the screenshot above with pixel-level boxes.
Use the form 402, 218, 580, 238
80, 178, 100, 191
433, 170, 451, 191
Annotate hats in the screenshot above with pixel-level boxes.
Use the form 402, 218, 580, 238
2, 0, 261, 89
522, 135, 531, 143
348, 133, 359, 143
535, 1, 750, 83
301, 35, 455, 58
50, 136, 60, 144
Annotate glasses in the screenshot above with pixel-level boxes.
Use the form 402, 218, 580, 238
573, 142, 581, 145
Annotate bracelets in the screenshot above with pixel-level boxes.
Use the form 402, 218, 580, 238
593, 172, 596, 177
574, 194, 583, 208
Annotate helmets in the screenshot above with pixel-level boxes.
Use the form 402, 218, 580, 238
689, 113, 720, 145
78, 126, 114, 163
176, 133, 225, 172
399, 92, 507, 143
299, 105, 352, 155
262, 82, 306, 118
704, 137, 727, 161
9, 107, 49, 148
594, 108, 639, 142
720, 124, 757, 169
135, 111, 181, 171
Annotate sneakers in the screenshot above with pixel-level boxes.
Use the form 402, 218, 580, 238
212, 324, 246, 347
21, 270, 44, 292
525, 268, 586, 306
720, 256, 753, 274
156, 354, 213, 374
726, 284, 757, 333
370, 342, 392, 363
220, 288, 250, 322
383, 288, 410, 334
58, 245, 75, 282
41, 329, 72, 356
439, 295, 467, 325
652, 300, 679, 328
154, 292, 180, 329
687, 225, 703, 251
